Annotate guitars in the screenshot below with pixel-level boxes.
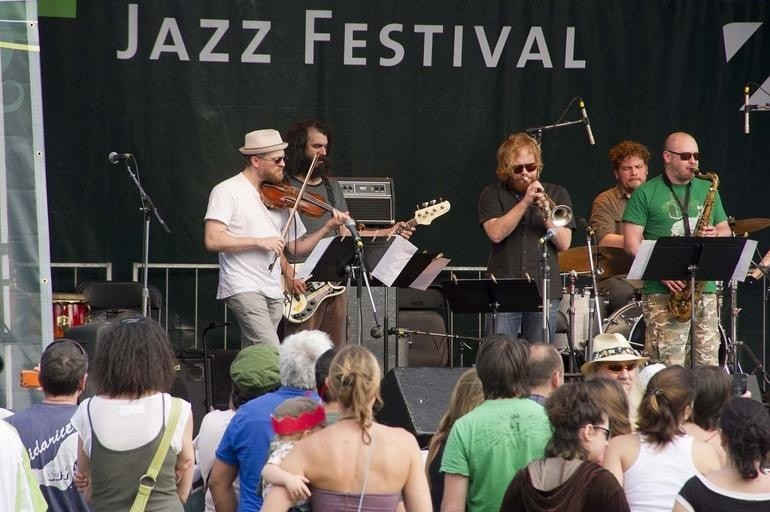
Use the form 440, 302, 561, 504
284, 197, 451, 323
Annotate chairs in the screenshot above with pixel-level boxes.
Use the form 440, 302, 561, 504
75, 280, 161, 339
397, 284, 451, 369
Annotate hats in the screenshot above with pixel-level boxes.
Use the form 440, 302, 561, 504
229, 341, 283, 393
579, 332, 650, 376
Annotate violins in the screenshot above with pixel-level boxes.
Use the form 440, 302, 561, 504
260, 181, 366, 231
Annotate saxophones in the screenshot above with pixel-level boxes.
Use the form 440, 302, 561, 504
667, 168, 719, 322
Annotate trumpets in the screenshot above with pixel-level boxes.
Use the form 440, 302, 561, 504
522, 176, 573, 226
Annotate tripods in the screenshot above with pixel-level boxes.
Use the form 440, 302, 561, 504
727, 279, 768, 380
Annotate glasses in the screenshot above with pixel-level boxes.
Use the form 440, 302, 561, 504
607, 362, 637, 373
256, 154, 290, 164
576, 425, 613, 441
511, 162, 538, 174
667, 150, 700, 162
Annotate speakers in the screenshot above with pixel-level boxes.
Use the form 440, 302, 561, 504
333, 176, 395, 226
377, 366, 469, 450
727, 375, 762, 403
170, 356, 211, 442
346, 282, 398, 378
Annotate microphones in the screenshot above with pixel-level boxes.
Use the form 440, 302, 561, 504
580, 218, 595, 237
579, 100, 596, 146
745, 85, 750, 133
345, 220, 363, 249
538, 228, 557, 244
109, 152, 130, 163
371, 325, 385, 339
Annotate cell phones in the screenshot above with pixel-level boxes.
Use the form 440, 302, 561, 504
732, 373, 747, 395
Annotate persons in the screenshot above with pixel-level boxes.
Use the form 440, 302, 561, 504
203, 128, 350, 350
589, 139, 651, 314
1, 311, 432, 511
277, 118, 417, 348
621, 131, 733, 370
478, 132, 578, 344
424, 333, 769, 511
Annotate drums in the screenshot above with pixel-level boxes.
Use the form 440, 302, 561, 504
558, 286, 593, 355
605, 299, 730, 368
28, 292, 90, 340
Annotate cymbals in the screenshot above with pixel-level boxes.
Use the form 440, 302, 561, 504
730, 217, 768, 235
557, 245, 632, 279
